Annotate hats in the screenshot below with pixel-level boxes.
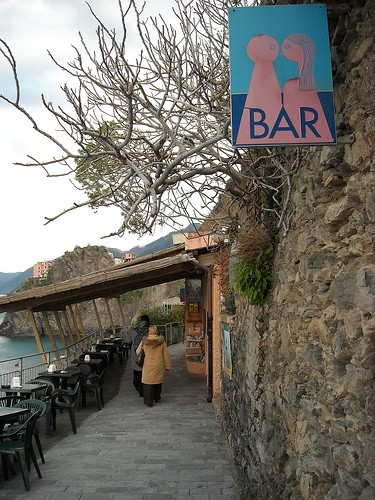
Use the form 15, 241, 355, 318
148, 325, 159, 335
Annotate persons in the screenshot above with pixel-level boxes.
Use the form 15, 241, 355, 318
136, 325, 170, 407
131, 312, 150, 397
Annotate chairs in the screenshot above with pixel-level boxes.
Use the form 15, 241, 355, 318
0, 366, 107, 492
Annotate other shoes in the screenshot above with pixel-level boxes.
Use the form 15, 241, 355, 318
147, 403, 153, 407
155, 396, 161, 402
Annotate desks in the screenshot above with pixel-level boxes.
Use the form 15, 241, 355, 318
0, 383, 47, 399
38, 371, 80, 414
71, 337, 129, 364
0, 406, 30, 419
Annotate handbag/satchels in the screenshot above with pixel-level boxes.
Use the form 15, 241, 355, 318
135, 351, 145, 368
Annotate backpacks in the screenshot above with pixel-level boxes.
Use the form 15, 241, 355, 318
134, 327, 148, 351
